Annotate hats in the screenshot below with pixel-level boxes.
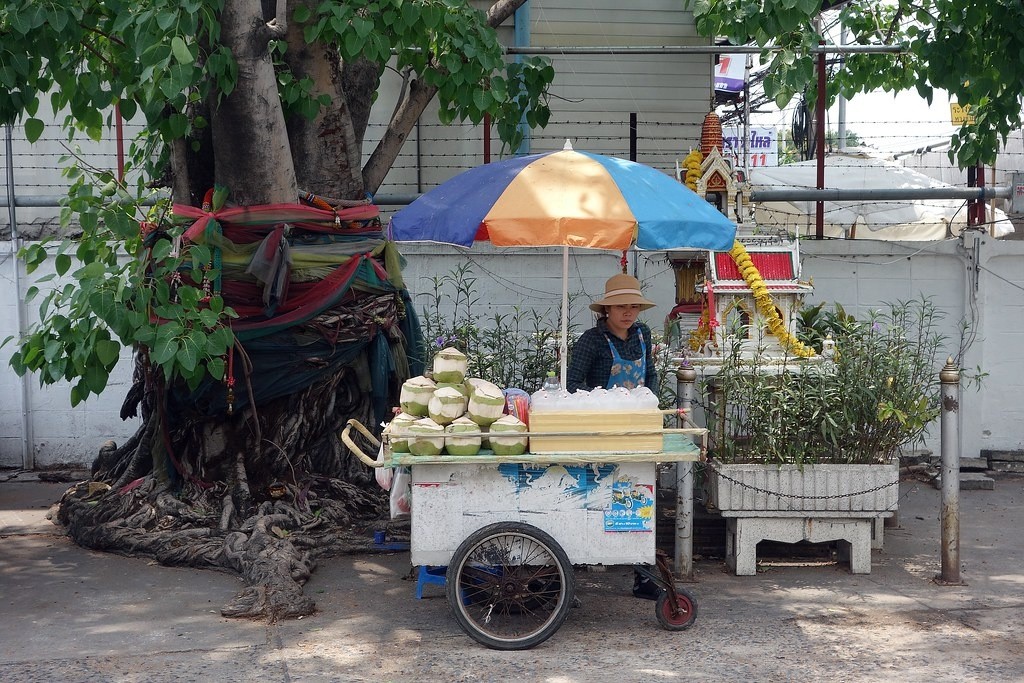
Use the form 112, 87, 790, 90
589, 274, 657, 314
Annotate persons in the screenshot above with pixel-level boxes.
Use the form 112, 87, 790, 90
566, 274, 660, 398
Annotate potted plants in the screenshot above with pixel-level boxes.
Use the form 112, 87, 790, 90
703, 294, 990, 514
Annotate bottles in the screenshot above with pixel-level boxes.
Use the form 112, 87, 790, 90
542, 372, 560, 391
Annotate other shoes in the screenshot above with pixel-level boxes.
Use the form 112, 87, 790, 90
633, 575, 664, 599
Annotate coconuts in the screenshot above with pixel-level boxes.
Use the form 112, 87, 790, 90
388, 346, 529, 456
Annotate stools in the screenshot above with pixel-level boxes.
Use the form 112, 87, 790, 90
415, 566, 503, 606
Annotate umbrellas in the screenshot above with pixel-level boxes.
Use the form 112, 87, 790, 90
750, 146, 1016, 240
387, 137, 737, 393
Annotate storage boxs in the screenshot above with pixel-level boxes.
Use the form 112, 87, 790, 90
528, 408, 664, 454
